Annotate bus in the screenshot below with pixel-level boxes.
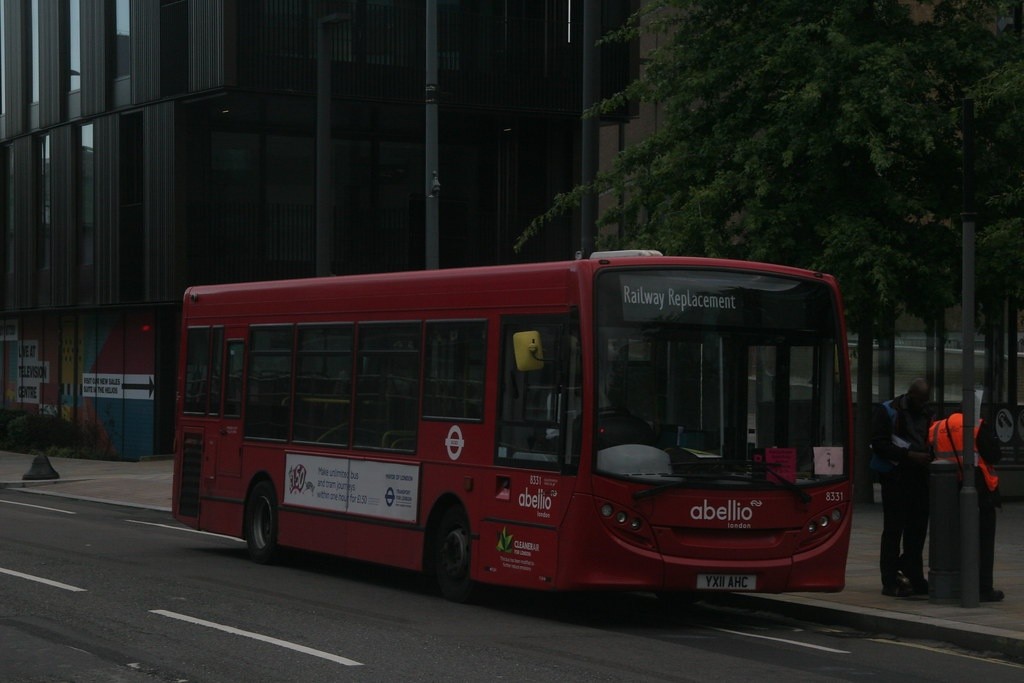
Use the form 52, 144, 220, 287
172, 251, 852, 602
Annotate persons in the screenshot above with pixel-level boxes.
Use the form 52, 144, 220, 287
927, 393, 1005, 602
870, 377, 934, 599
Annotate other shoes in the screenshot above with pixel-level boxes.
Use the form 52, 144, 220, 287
980, 589, 1004, 602
879, 570, 914, 597
899, 553, 929, 594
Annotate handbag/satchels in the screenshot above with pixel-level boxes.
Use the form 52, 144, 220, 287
864, 402, 896, 479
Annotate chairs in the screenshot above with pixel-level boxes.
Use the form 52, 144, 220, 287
187, 366, 484, 456
